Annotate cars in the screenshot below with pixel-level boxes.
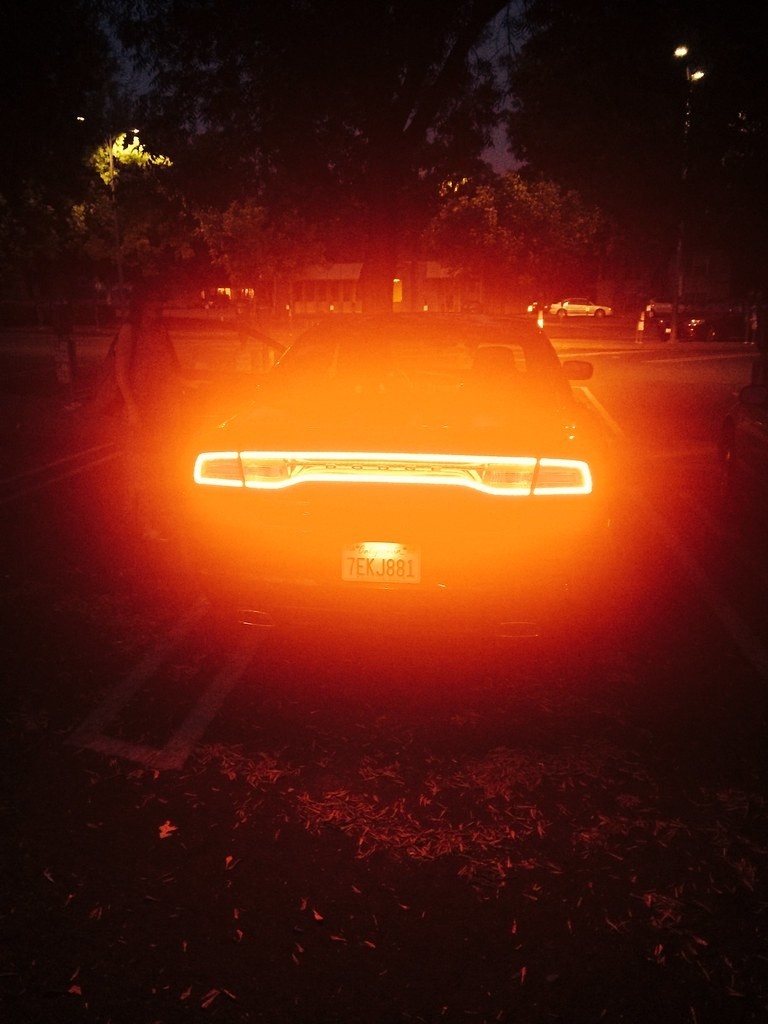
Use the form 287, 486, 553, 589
655, 303, 750, 341
144, 306, 596, 641
645, 296, 685, 317
548, 298, 613, 319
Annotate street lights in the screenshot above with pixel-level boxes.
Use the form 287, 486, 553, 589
671, 44, 706, 298
